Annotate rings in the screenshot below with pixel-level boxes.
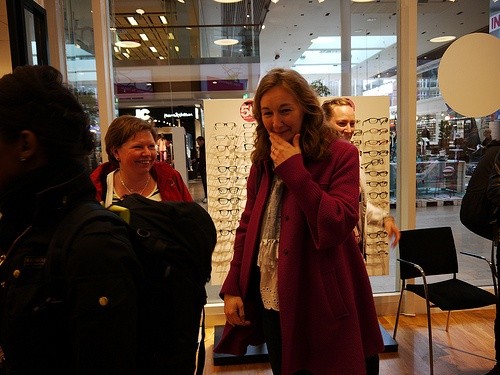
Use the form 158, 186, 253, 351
272, 148, 275, 153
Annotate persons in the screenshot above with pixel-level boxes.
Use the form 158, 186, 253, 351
157, 133, 172, 162
191, 136, 207, 203
457, 127, 500, 374
0, 64, 138, 375
219, 68, 386, 375
89, 114, 194, 210
321, 97, 404, 260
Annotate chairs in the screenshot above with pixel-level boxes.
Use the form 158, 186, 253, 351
393, 226, 499, 375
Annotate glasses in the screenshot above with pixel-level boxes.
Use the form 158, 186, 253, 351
366, 250, 388, 258
367, 192, 388, 199
366, 231, 388, 238
364, 171, 389, 177
213, 122, 236, 130
365, 158, 383, 168
350, 118, 390, 157
215, 132, 256, 236
242, 122, 258, 128
368, 241, 389, 248
370, 201, 389, 208
366, 182, 387, 187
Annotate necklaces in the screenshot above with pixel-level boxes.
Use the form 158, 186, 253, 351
118, 171, 150, 196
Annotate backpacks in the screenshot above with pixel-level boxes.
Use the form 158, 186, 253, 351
38, 193, 217, 375
482, 145, 500, 241
